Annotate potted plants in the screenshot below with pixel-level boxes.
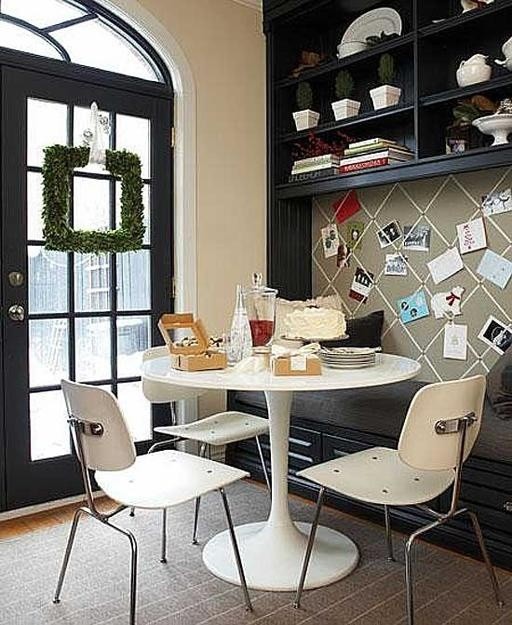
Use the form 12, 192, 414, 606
292, 51, 402, 132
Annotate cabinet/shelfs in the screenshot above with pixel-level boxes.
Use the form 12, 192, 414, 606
266, 2, 511, 200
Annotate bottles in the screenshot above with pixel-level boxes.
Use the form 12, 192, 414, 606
226, 284, 252, 362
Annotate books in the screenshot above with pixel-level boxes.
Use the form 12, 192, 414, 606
286, 138, 412, 184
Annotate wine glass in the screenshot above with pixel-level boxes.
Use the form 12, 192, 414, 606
472, 113, 512, 146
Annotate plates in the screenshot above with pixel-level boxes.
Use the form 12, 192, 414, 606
432, 19, 444, 23
339, 8, 403, 44
319, 348, 376, 368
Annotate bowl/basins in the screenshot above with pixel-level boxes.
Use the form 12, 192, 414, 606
455, 64, 491, 88
336, 40, 366, 56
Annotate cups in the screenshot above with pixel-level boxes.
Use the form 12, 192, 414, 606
245, 288, 278, 346
